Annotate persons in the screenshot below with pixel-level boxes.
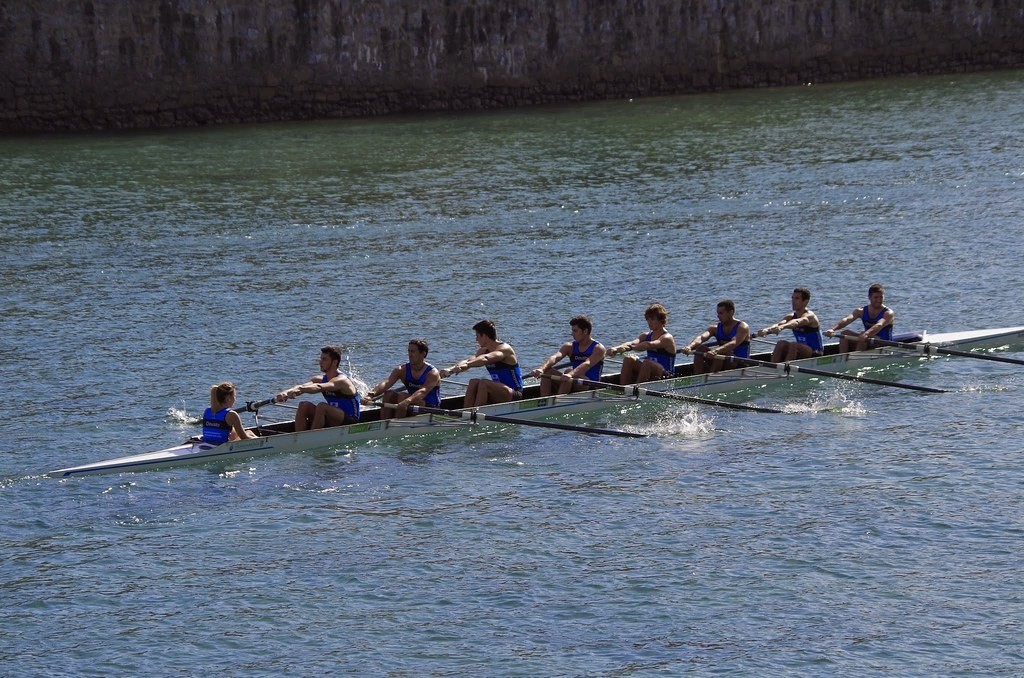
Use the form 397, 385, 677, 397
362, 339, 441, 420
825, 283, 893, 353
682, 300, 750, 374
203, 383, 257, 444
757, 288, 823, 363
278, 346, 360, 431
607, 303, 675, 385
531, 315, 606, 397
439, 319, 523, 408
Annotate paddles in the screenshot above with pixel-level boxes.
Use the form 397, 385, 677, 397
674, 327, 779, 354
369, 368, 459, 403
821, 330, 1024, 368
679, 348, 955, 394
184, 391, 296, 425
360, 399, 650, 439
521, 350, 628, 379
536, 372, 808, 416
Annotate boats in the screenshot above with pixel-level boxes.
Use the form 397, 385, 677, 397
44, 323, 1024, 484
